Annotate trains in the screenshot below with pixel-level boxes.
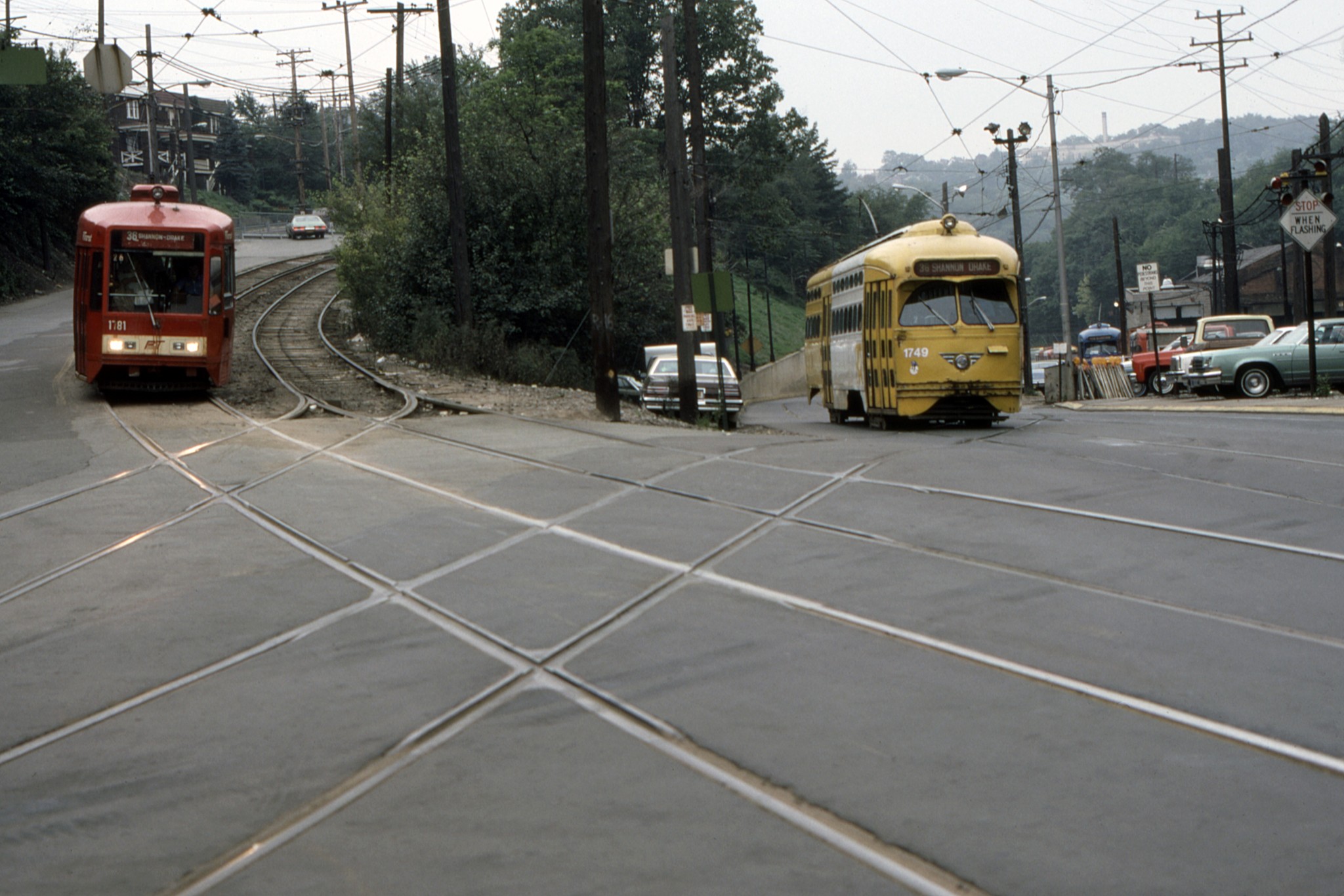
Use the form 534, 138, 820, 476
72, 184, 235, 391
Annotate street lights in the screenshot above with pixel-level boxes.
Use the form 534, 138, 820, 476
338, 126, 365, 184
147, 79, 213, 186
254, 134, 307, 214
982, 122, 1034, 396
935, 68, 1076, 402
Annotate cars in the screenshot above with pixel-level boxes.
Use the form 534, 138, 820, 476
285, 215, 328, 239
1163, 318, 1344, 399
617, 341, 744, 430
1032, 359, 1066, 396
1119, 313, 1274, 396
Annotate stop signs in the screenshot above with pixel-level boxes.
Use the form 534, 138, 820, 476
1279, 189, 1337, 252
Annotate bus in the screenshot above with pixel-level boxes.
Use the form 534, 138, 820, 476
1079, 322, 1124, 361
803, 213, 1028, 432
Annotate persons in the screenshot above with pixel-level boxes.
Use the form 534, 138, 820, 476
118, 258, 144, 293
